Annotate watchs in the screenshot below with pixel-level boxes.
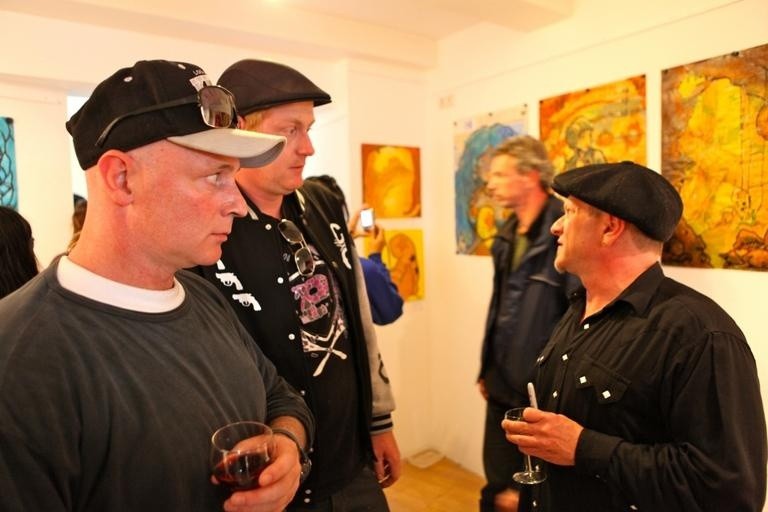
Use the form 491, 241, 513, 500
272, 428, 312, 484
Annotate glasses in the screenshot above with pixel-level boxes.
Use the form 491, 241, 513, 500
95, 85, 239, 147
277, 217, 316, 277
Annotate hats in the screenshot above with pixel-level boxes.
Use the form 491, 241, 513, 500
553, 161, 684, 241
217, 61, 332, 116
66, 59, 287, 168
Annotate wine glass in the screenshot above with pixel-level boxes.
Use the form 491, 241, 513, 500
503, 407, 548, 486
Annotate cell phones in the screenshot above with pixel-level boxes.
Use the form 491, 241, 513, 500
360, 208, 376, 233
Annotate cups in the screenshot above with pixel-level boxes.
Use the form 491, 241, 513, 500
209, 421, 278, 491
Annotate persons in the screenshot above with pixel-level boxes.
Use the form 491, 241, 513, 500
72, 198, 88, 234
0, 205, 44, 300
501, 160, 768, 512
1, 58, 316, 512
305, 175, 404, 327
473, 138, 582, 512
181, 60, 405, 512
